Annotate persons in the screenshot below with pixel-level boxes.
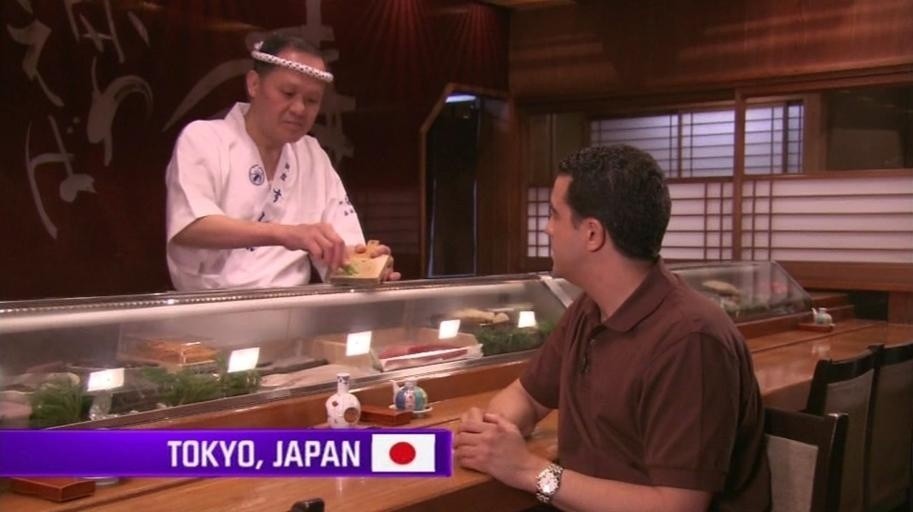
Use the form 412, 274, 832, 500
164, 33, 400, 288
451, 145, 771, 511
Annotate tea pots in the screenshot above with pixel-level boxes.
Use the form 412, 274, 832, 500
388, 377, 428, 410
810, 305, 833, 327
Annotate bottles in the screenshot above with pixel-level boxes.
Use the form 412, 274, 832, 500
323, 373, 362, 429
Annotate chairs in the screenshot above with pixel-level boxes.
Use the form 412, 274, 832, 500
762, 342, 913, 512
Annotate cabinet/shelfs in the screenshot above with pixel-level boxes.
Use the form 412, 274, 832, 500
0, 260, 814, 431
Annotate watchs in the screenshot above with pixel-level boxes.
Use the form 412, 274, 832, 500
535, 463, 562, 504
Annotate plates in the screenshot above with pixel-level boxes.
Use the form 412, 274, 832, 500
250, 372, 296, 389
0, 370, 83, 404
